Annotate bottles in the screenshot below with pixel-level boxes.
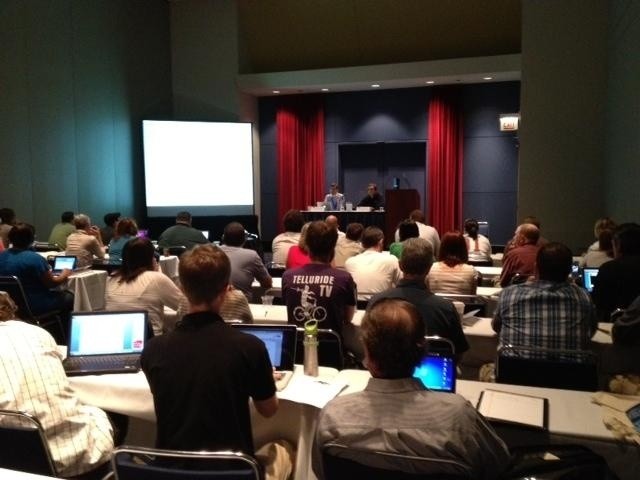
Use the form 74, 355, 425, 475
304, 325, 319, 378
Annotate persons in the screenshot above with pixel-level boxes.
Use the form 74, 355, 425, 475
323, 182, 347, 211
356, 182, 384, 211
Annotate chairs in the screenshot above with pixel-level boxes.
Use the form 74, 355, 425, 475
1, 239, 640, 479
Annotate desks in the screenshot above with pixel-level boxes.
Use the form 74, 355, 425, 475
300, 210, 387, 252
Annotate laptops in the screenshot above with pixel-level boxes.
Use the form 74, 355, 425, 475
51, 256, 77, 276
231, 324, 297, 392
317, 202, 334, 211
412, 336, 455, 392
136, 229, 148, 237
198, 229, 209, 240
62, 310, 148, 377
582, 268, 599, 293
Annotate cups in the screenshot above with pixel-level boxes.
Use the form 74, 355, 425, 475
261, 295, 275, 306
452, 302, 466, 328
379, 207, 383, 211
152, 240, 157, 248
346, 202, 352, 211
340, 203, 344, 211
